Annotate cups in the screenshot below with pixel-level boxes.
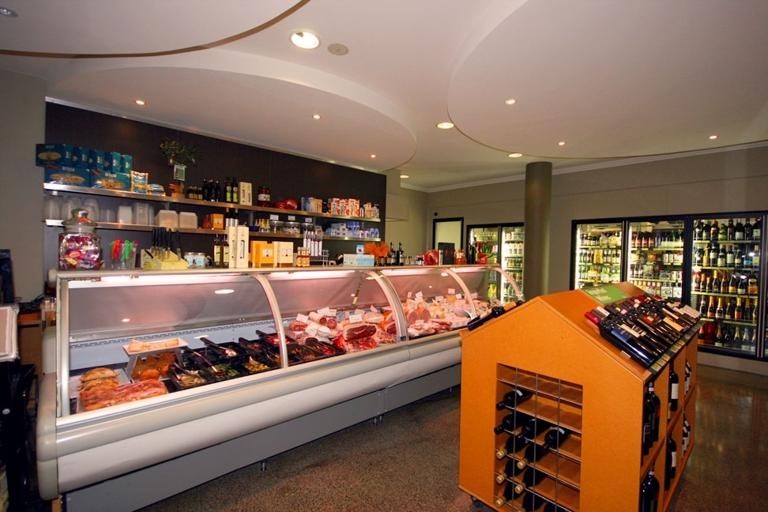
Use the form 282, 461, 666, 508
110, 239, 139, 270
42, 195, 154, 227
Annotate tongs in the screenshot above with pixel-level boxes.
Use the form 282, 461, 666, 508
199, 337, 232, 359
263, 348, 280, 362
310, 338, 328, 352
170, 360, 201, 385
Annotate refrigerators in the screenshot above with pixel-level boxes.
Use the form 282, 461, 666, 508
569, 217, 688, 303
466, 224, 524, 303
688, 213, 768, 361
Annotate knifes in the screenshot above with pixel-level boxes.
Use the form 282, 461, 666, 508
151, 226, 182, 262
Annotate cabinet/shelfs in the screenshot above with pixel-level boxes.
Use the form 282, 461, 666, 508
577, 222, 755, 355
452, 281, 705, 511
41, 184, 382, 245
56, 260, 522, 498
471, 238, 522, 274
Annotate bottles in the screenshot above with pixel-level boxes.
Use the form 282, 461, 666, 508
346, 221, 380, 238
692, 219, 762, 348
378, 242, 405, 266
186, 176, 239, 202
639, 354, 694, 511
258, 185, 272, 207
403, 256, 425, 266
57, 208, 104, 272
473, 227, 524, 301
184, 251, 209, 269
495, 388, 534, 411
584, 292, 704, 370
322, 202, 329, 215
496, 411, 572, 512
254, 219, 314, 235
212, 232, 229, 267
224, 208, 240, 230
576, 229, 621, 288
296, 247, 310, 267
630, 226, 682, 298
